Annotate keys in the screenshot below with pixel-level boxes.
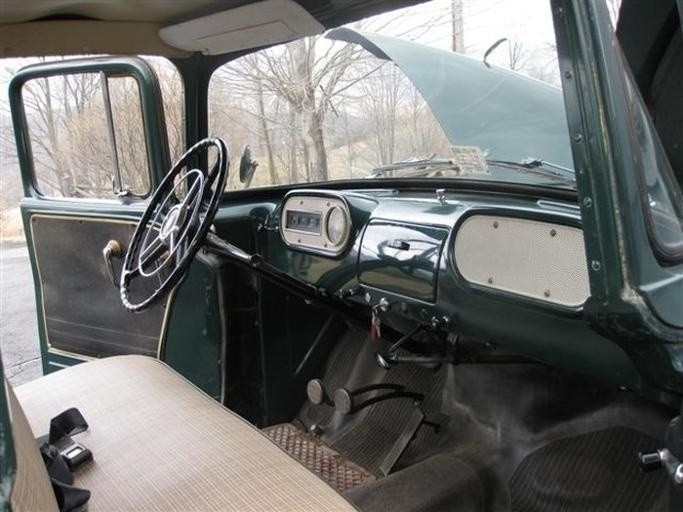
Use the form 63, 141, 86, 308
370, 303, 386, 343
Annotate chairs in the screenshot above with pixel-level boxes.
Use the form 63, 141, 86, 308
0, 355, 360, 511
